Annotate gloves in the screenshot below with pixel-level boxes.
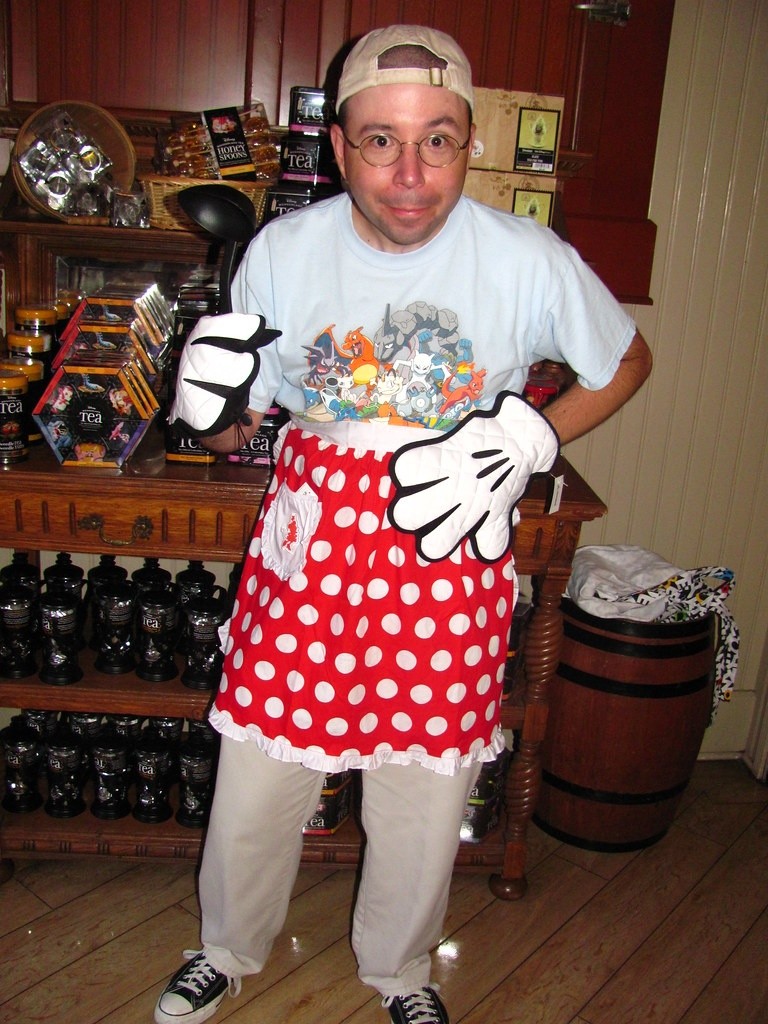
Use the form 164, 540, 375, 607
167, 313, 282, 438
387, 390, 563, 563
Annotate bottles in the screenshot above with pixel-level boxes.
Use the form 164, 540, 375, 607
0, 551, 241, 690
1, 710, 220, 829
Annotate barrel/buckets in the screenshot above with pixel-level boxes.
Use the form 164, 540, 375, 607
530, 596, 715, 854
11, 98, 139, 223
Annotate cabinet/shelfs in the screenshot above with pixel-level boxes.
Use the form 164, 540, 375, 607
1, 218, 608, 900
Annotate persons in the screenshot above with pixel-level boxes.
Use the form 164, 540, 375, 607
153, 23, 652, 1024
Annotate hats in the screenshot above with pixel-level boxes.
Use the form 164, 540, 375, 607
335, 25, 474, 123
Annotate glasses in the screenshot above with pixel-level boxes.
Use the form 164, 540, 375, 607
342, 128, 472, 168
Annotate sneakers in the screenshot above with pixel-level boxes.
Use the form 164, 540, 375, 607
381, 981, 449, 1024
153, 947, 242, 1024
